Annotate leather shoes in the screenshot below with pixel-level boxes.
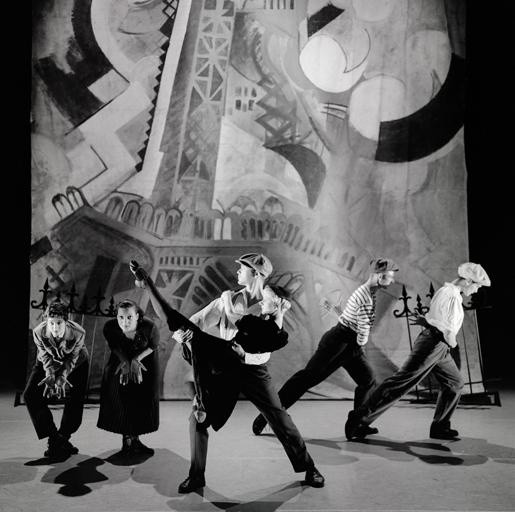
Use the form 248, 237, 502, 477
305, 469, 324, 488
253, 413, 267, 435
179, 477, 206, 494
430, 428, 459, 440
116, 437, 154, 459
345, 423, 378, 439
42, 433, 79, 457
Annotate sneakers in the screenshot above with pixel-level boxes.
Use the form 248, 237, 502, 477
129, 259, 148, 288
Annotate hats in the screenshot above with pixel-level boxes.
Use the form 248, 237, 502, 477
369, 258, 399, 273
235, 252, 273, 278
457, 262, 490, 287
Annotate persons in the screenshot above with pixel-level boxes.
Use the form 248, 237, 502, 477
250, 256, 400, 436
171, 253, 326, 494
344, 260, 495, 440
19, 298, 91, 464
96, 299, 163, 453
126, 258, 292, 431
